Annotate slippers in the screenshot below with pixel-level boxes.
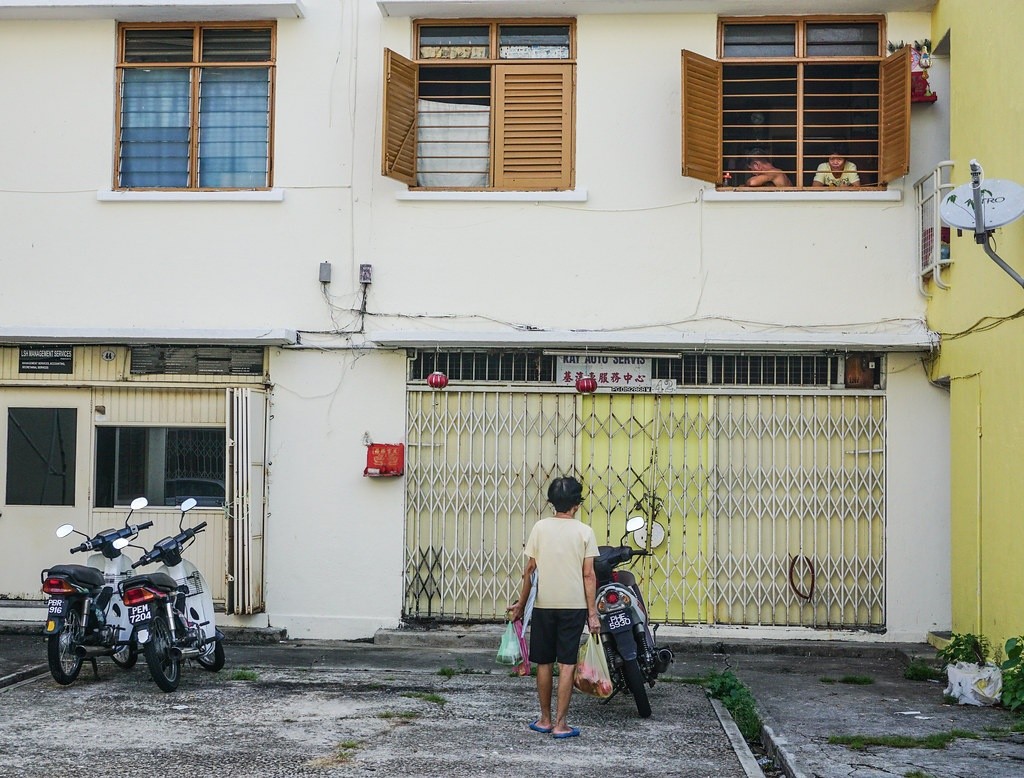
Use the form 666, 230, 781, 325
529, 720, 551, 733
552, 727, 580, 738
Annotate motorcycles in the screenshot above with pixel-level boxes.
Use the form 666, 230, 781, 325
591, 515, 675, 717
114, 496, 224, 692
38, 497, 153, 685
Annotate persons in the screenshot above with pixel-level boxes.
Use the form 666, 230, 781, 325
507, 477, 601, 737
738, 148, 793, 187
812, 144, 861, 187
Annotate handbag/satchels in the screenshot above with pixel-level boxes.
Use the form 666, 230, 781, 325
495, 621, 524, 667
573, 631, 613, 699
511, 619, 530, 676
